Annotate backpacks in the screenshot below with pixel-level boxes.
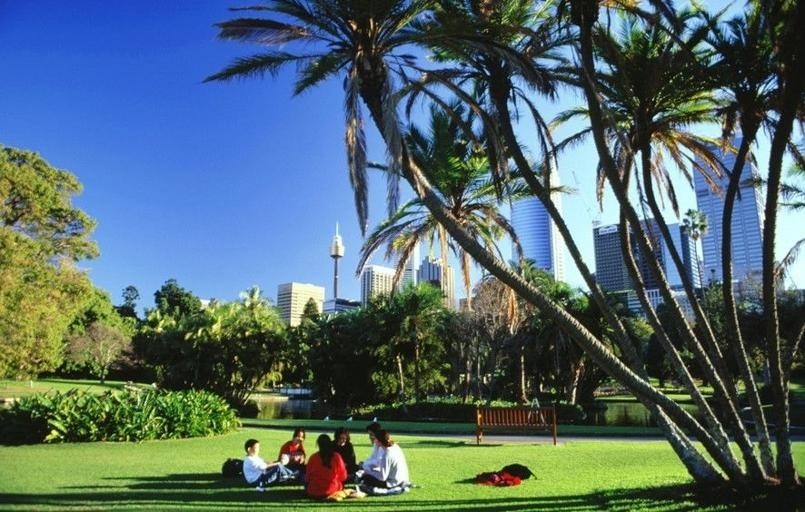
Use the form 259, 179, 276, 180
476, 470, 520, 487
503, 463, 538, 480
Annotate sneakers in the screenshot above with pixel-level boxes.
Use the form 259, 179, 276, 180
279, 475, 296, 484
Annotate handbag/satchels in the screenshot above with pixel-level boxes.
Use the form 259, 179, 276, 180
222, 458, 243, 478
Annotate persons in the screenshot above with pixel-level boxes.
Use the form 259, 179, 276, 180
304, 433, 348, 499
360, 421, 383, 471
330, 426, 356, 474
359, 432, 409, 490
278, 425, 306, 474
241, 439, 293, 487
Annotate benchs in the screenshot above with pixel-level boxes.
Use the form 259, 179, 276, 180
472, 404, 559, 446
